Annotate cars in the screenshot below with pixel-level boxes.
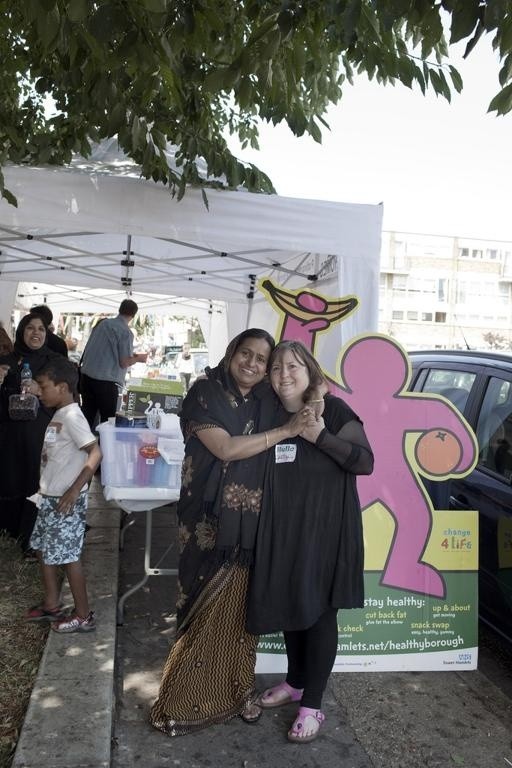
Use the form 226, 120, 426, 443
406, 350, 511, 649
132, 348, 209, 379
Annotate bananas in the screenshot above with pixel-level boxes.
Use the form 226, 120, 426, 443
262, 280, 358, 323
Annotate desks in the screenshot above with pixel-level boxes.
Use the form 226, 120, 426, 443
103, 485, 180, 628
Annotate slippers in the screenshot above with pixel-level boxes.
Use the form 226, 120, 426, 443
240, 703, 264, 723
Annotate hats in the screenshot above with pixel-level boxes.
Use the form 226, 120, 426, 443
50, 356, 79, 402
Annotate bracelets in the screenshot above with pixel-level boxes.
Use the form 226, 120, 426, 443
264, 432, 271, 451
307, 398, 324, 403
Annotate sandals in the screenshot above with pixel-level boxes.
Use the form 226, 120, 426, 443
257, 680, 304, 708
288, 707, 325, 743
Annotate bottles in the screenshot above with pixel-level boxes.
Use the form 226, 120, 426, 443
20, 362, 32, 399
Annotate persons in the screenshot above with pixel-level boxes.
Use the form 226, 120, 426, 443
23, 359, 103, 635
0, 303, 80, 563
77, 299, 149, 476
242, 339, 374, 744
174, 342, 195, 392
147, 327, 328, 737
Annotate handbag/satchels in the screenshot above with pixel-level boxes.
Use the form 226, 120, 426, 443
9, 390, 39, 420
78, 366, 82, 394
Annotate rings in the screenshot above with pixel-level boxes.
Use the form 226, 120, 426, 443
301, 410, 308, 417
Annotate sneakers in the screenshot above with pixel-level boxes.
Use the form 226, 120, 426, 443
50, 608, 96, 634
23, 598, 65, 623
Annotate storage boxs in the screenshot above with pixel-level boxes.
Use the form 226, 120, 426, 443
96, 418, 184, 490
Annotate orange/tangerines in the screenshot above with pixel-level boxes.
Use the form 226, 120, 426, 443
416, 428, 464, 476
